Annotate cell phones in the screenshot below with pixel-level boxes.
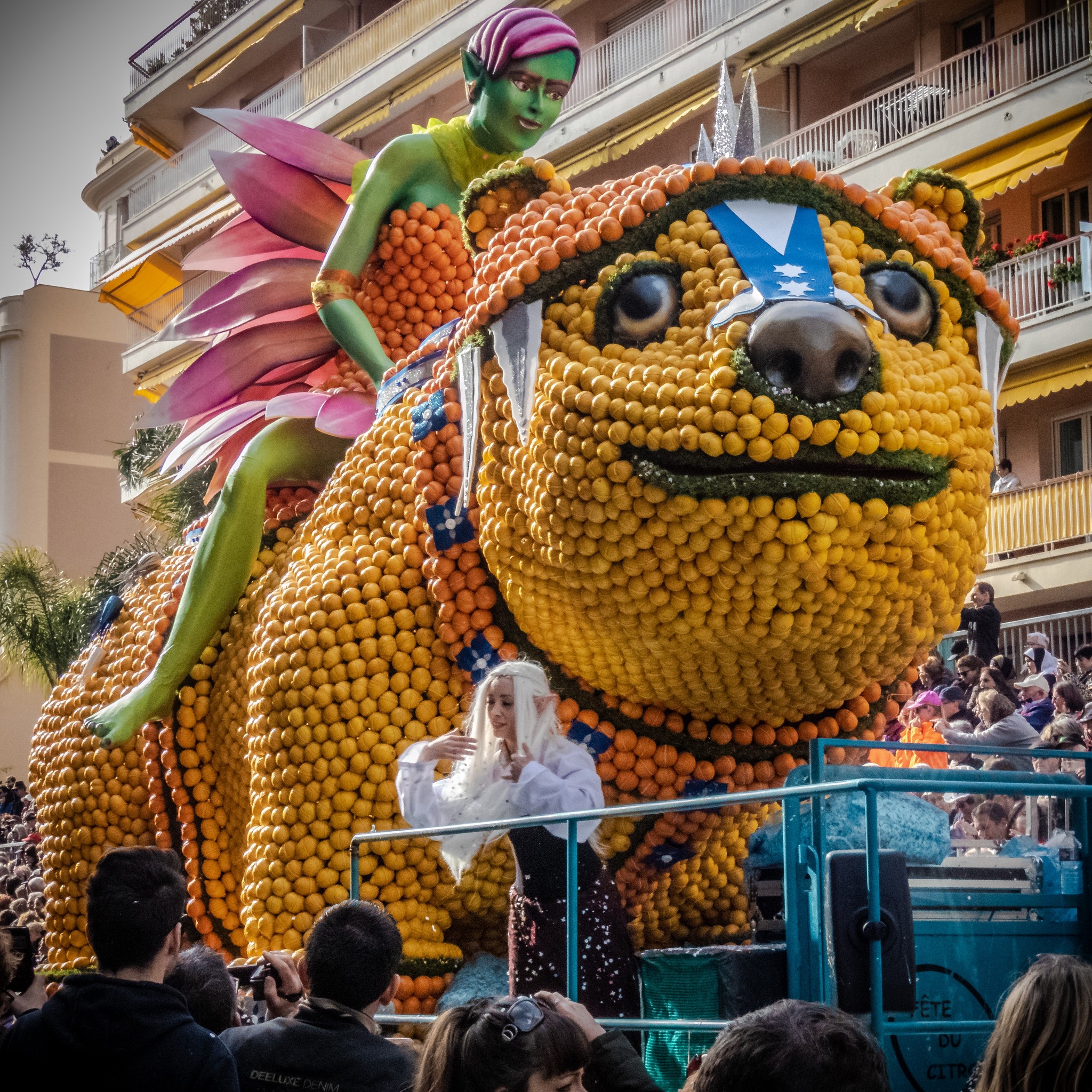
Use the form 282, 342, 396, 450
1057, 659, 1064, 665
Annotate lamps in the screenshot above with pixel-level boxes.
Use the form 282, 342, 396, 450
1010, 571, 1025, 581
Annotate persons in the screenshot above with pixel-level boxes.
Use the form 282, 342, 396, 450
991, 458, 1022, 561
976, 953, 1092, 1092
868, 633, 1092, 858
961, 582, 1002, 667
81, 7, 577, 753
395, 661, 640, 1020
0, 773, 890, 1092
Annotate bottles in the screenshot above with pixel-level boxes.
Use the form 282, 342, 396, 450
1059, 831, 1083, 895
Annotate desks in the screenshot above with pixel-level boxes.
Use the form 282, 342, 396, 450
788, 149, 846, 172
874, 83, 951, 138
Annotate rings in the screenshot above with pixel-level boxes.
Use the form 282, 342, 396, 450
551, 998, 561, 1007
564, 997, 571, 1000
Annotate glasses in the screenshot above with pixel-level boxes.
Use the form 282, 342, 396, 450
1025, 641, 1040, 646
498, 994, 554, 1054
956, 796, 975, 810
956, 668, 972, 677
686, 1053, 707, 1079
1017, 691, 1041, 706
1051, 695, 1060, 701
1031, 756, 1049, 761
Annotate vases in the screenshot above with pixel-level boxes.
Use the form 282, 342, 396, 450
1017, 244, 1063, 276
984, 268, 1011, 291
1068, 279, 1085, 306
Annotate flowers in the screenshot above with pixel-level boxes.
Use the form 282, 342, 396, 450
973, 232, 1082, 291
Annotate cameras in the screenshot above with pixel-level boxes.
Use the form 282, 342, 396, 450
931, 722, 935, 728
227, 963, 282, 1000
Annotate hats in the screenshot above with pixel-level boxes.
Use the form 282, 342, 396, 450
1054, 732, 1087, 751
944, 766, 978, 803
938, 686, 965, 700
947, 639, 968, 661
1076, 702, 1092, 723
907, 690, 942, 710
1028, 741, 1054, 750
1013, 673, 1050, 694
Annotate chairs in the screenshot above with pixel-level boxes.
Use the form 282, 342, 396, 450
834, 129, 878, 168
